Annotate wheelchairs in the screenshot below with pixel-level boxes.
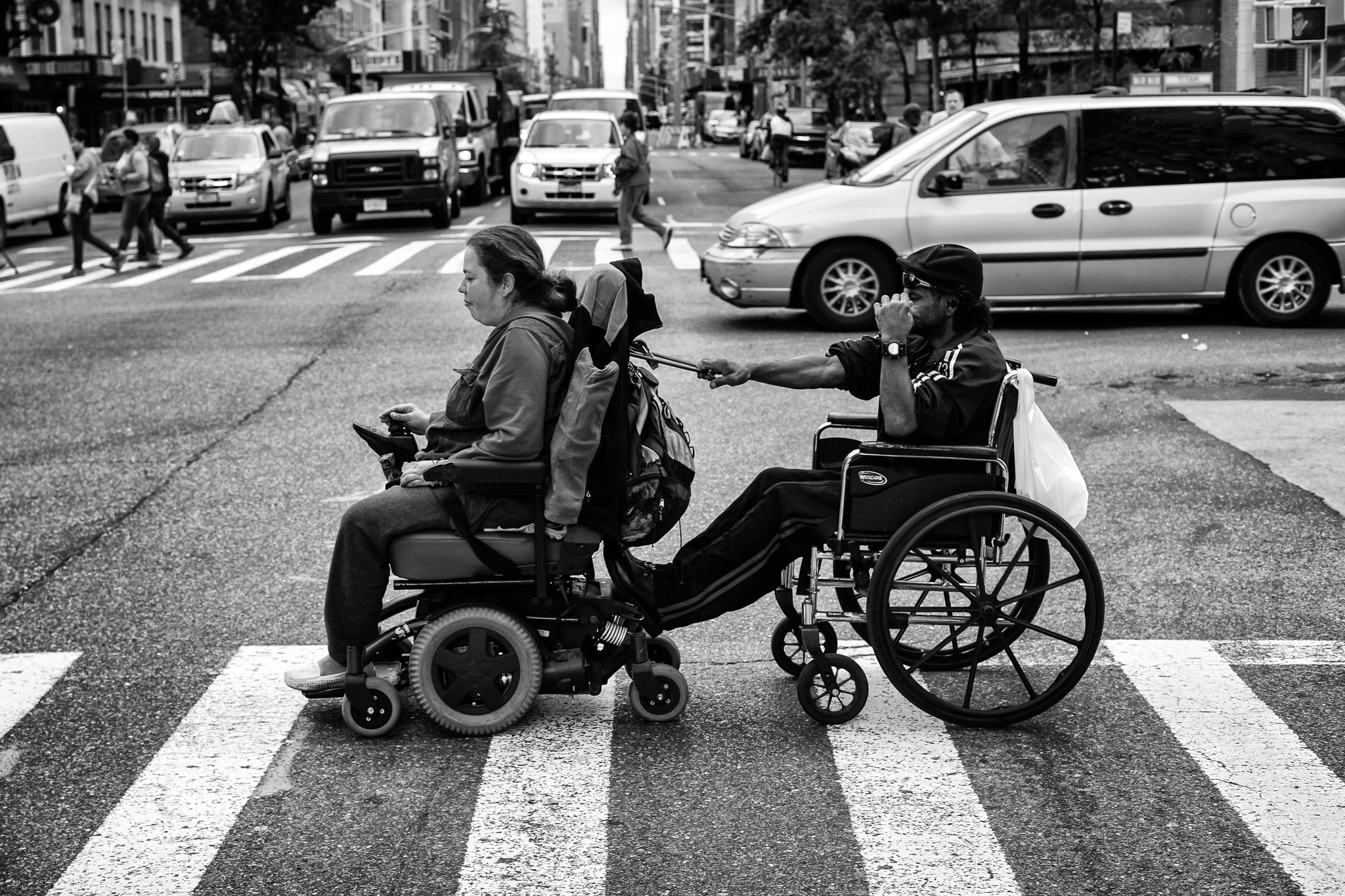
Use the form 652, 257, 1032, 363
770, 365, 1103, 725
302, 258, 692, 735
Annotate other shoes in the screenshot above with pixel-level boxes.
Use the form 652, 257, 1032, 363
100, 249, 126, 270
127, 253, 150, 262
612, 243, 632, 250
662, 226, 673, 248
116, 254, 127, 272
61, 267, 86, 280
176, 244, 195, 259
284, 662, 377, 691
138, 251, 162, 270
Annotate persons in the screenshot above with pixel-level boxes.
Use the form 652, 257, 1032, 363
602, 244, 1007, 639
610, 89, 1016, 251
0, 115, 312, 279
285, 225, 577, 693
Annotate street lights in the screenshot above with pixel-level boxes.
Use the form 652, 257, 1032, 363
454, 27, 493, 70
634, 62, 684, 127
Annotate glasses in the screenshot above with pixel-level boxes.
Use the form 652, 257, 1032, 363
902, 272, 952, 292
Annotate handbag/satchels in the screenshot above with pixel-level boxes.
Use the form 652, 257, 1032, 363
64, 178, 82, 215
79, 150, 117, 195
1012, 369, 1088, 539
759, 144, 774, 162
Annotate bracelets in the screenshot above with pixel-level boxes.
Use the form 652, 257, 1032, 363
431, 457, 444, 465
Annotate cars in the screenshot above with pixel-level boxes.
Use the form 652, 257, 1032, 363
824, 120, 893, 180
739, 119, 760, 158
509, 111, 648, 226
689, 91, 743, 142
87, 123, 314, 233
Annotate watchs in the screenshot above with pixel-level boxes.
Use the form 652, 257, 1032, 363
879, 338, 908, 359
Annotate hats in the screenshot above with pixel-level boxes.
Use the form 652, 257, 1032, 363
895, 244, 983, 302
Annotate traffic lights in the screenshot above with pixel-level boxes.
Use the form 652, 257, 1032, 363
1290, 5, 1329, 43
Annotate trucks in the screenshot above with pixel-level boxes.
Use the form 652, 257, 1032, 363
380, 64, 522, 206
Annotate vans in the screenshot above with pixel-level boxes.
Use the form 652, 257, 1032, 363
521, 88, 651, 135
0, 113, 77, 237
701, 87, 1345, 330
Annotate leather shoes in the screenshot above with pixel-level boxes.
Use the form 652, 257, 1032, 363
602, 540, 665, 638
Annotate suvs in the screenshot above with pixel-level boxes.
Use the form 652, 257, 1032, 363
753, 109, 839, 165
308, 91, 460, 236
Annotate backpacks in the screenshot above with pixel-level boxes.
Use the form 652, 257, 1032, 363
130, 149, 165, 192
855, 124, 896, 166
618, 368, 695, 549
